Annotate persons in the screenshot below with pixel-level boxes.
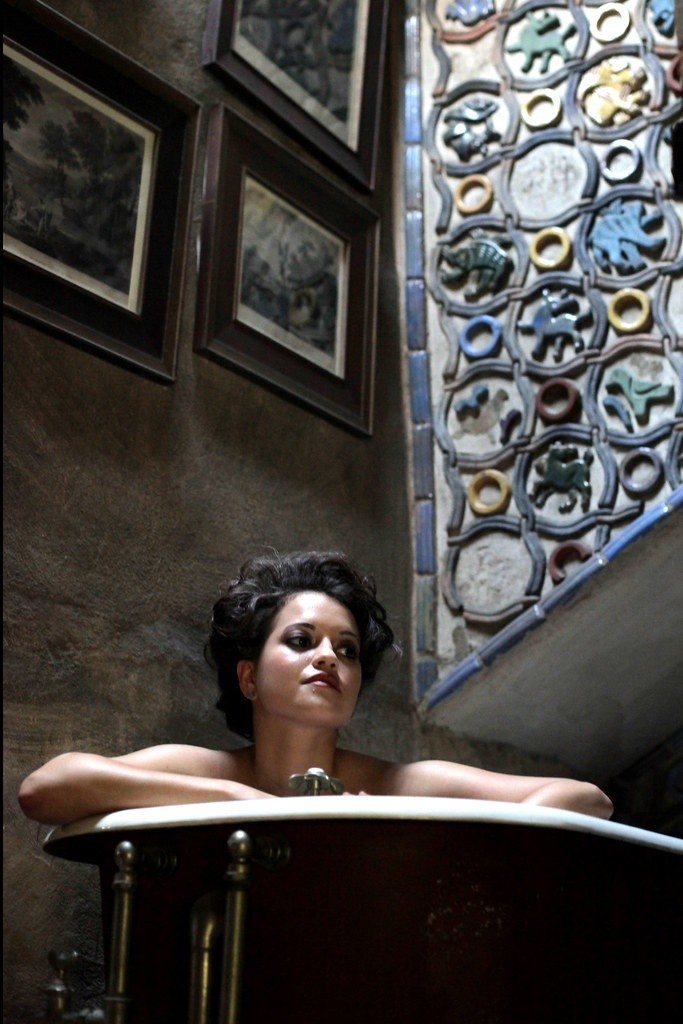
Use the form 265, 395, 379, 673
17, 549, 615, 826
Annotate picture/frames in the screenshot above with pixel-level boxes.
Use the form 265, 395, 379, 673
199, 0, 391, 196
192, 103, 383, 442
0, 0, 187, 387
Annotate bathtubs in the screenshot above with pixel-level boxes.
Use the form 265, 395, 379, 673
40, 794, 683, 1024
